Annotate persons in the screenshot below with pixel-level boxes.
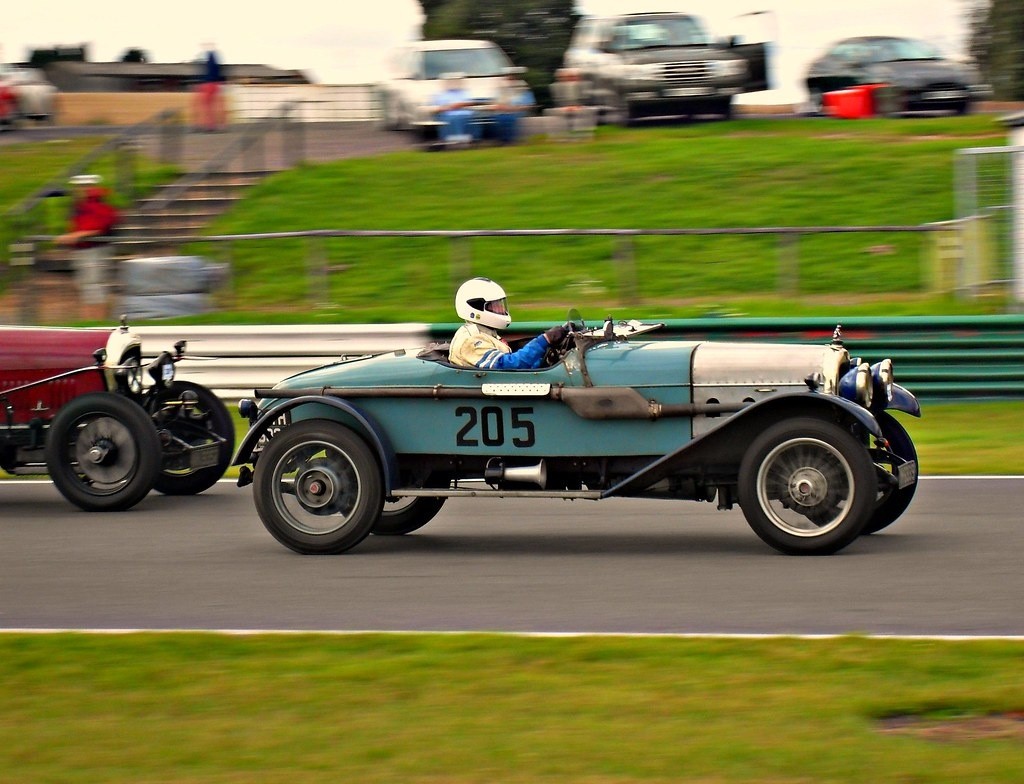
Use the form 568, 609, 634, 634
0, 46, 228, 131
449, 277, 575, 370
432, 58, 599, 150
51, 174, 120, 318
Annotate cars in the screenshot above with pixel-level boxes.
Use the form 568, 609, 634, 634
804, 35, 995, 119
378, 33, 537, 145
228, 314, 922, 558
0, 315, 237, 513
562, 12, 769, 125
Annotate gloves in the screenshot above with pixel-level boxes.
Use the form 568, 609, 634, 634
544, 325, 566, 344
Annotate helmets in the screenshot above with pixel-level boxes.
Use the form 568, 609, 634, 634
455, 277, 511, 329
